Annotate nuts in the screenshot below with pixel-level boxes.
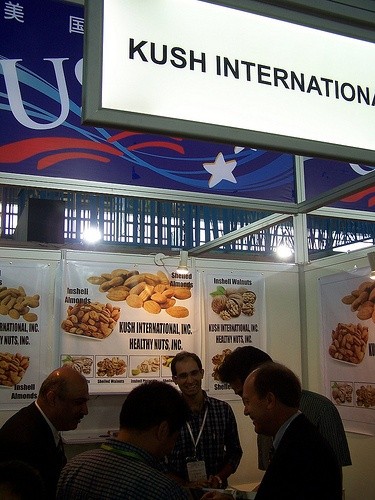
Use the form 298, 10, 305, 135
328, 281, 375, 409
0, 268, 257, 387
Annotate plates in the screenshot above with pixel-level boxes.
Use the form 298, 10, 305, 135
328, 344, 366, 367
0, 368, 32, 388
62, 319, 117, 339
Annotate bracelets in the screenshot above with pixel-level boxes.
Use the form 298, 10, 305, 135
215, 475, 222, 488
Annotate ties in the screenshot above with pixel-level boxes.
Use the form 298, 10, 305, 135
269, 444, 275, 466
57, 434, 65, 459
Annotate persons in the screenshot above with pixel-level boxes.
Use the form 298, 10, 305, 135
56, 381, 191, 500
0, 368, 89, 500
219, 345, 352, 492
198, 363, 342, 500
159, 352, 242, 500
